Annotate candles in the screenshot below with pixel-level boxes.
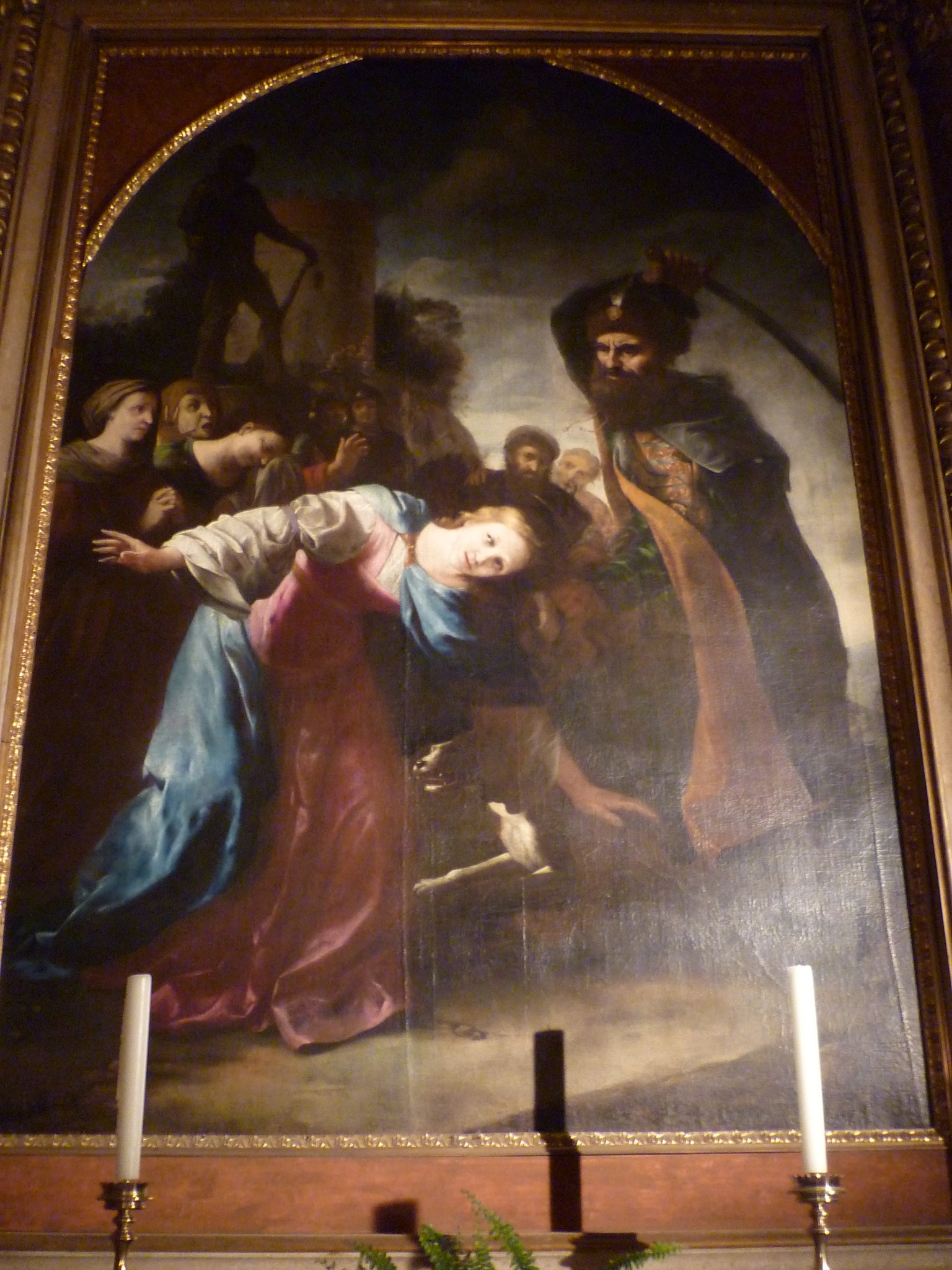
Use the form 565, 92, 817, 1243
788, 966, 828, 1158
116, 974, 152, 1181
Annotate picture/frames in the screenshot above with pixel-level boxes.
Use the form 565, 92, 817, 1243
0, 0, 952, 1162
